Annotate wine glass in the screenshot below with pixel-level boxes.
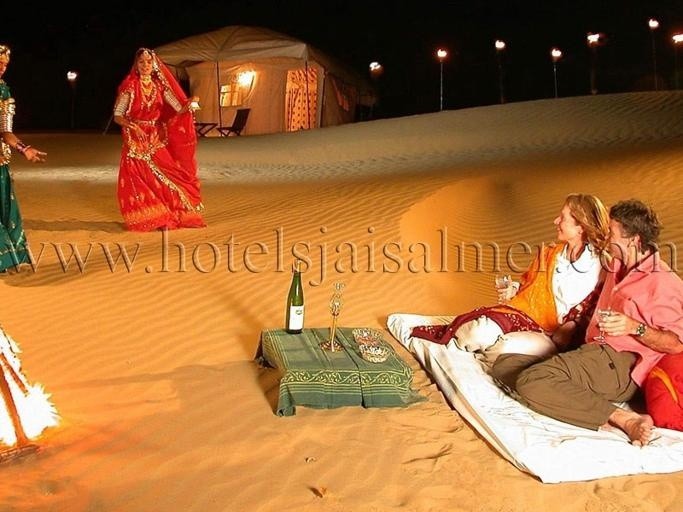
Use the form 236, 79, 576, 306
592, 306, 614, 345
320, 282, 346, 352
493, 272, 511, 305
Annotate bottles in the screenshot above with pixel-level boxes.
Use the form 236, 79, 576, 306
285, 258, 305, 336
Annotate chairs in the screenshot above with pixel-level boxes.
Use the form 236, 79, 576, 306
218, 107, 251, 139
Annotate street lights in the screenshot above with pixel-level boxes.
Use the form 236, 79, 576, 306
551, 48, 562, 98
367, 61, 384, 119
649, 18, 659, 91
67, 71, 78, 128
495, 40, 505, 104
437, 49, 447, 111
587, 34, 600, 96
672, 34, 683, 89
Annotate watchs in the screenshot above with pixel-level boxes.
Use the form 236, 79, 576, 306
634, 323, 647, 338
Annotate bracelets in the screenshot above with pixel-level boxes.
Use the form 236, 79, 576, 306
14, 139, 29, 156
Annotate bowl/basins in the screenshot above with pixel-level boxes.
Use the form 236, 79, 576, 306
353, 327, 392, 365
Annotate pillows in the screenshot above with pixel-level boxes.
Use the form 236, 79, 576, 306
643, 351, 683, 433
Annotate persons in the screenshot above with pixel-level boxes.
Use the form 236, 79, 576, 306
409, 192, 612, 369
0, 323, 61, 468
491, 199, 683, 449
111, 48, 208, 230
1, 46, 47, 272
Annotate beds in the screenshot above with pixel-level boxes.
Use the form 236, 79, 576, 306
192, 121, 218, 138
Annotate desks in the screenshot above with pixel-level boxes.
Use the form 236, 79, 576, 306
251, 326, 427, 420
